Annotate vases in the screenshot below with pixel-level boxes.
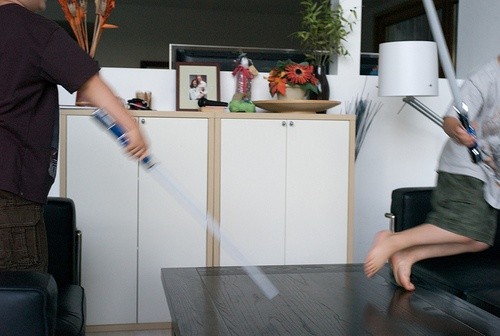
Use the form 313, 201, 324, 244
277, 83, 308, 100
308, 63, 330, 114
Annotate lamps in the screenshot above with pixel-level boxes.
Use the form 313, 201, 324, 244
377, 40, 495, 159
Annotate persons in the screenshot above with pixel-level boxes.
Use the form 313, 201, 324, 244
190, 75, 207, 99
365, 53, 500, 291
0, 0, 150, 274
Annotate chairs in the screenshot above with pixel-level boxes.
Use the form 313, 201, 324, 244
0, 196, 87, 336
383, 183, 500, 336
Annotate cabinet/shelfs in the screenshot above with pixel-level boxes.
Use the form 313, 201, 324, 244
59, 108, 214, 331
212, 113, 356, 266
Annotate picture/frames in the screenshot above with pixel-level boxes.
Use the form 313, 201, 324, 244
176, 61, 221, 112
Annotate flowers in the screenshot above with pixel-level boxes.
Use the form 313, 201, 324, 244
267, 58, 322, 98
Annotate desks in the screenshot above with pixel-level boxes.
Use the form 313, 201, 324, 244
162, 263, 487, 336
